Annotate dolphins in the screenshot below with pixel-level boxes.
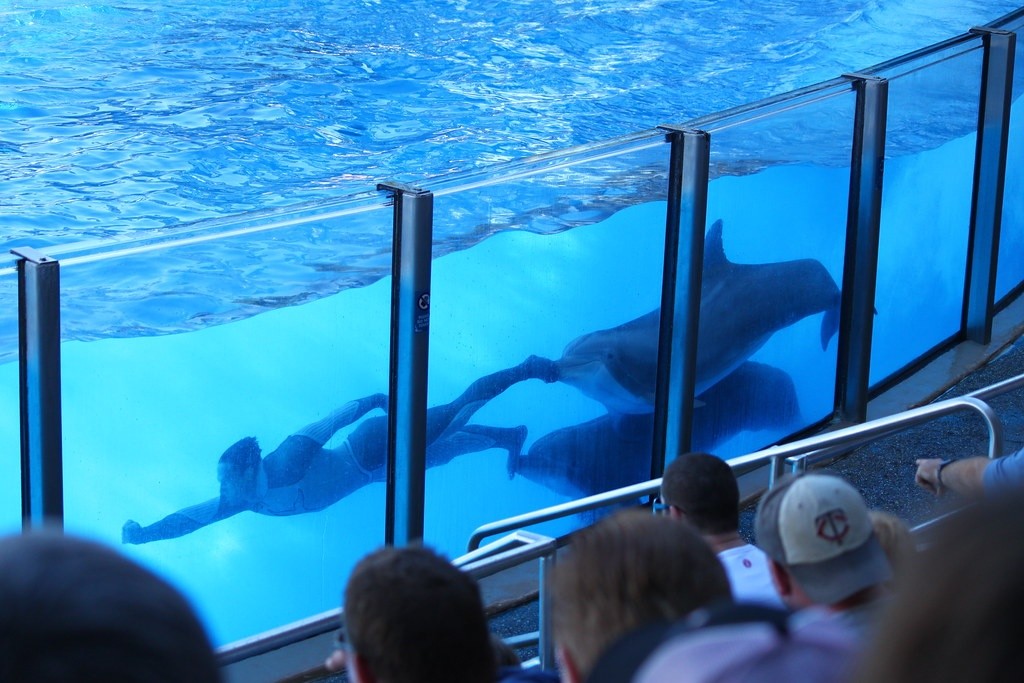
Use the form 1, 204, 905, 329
517, 362, 801, 501
554, 218, 879, 416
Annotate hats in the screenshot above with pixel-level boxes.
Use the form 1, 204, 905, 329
753, 468, 894, 604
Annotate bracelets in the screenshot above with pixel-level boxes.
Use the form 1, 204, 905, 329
936, 458, 958, 488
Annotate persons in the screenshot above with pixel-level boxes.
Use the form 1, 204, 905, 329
0, 445, 1024, 683
122, 356, 559, 545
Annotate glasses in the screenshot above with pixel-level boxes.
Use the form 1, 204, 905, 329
651, 495, 690, 518
331, 629, 362, 653
